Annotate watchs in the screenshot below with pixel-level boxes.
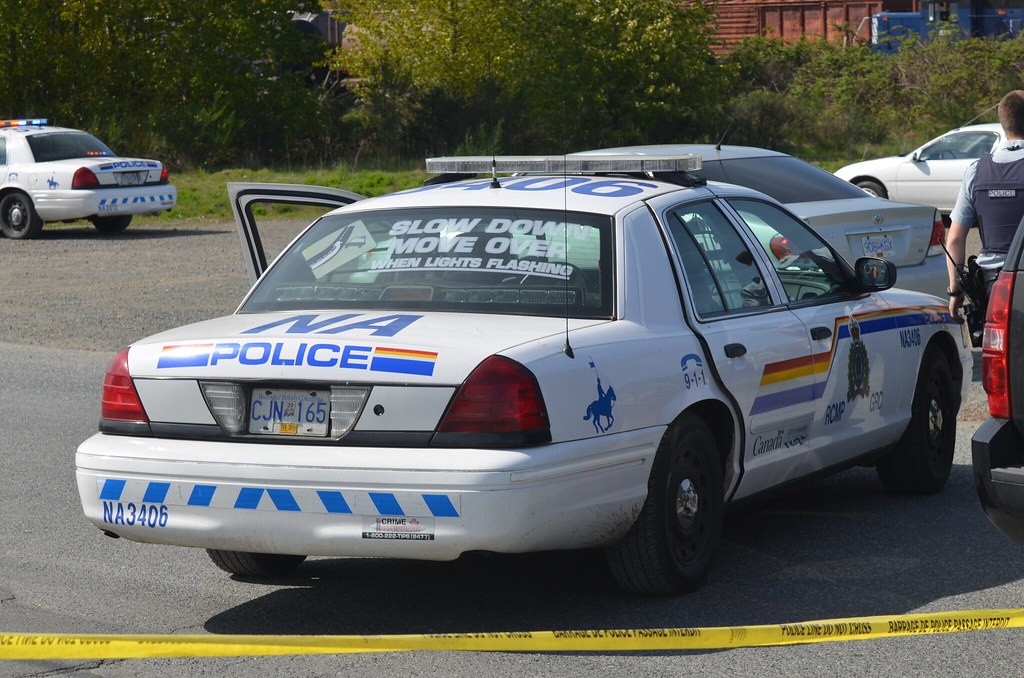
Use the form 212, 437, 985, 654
945, 287, 962, 296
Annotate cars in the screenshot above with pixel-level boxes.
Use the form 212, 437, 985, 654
0, 118, 179, 240
511, 141, 955, 305
832, 122, 1008, 215
969, 218, 1023, 548
75, 152, 975, 603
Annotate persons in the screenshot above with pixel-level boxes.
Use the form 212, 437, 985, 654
871, 263, 876, 279
944, 90, 1024, 346
